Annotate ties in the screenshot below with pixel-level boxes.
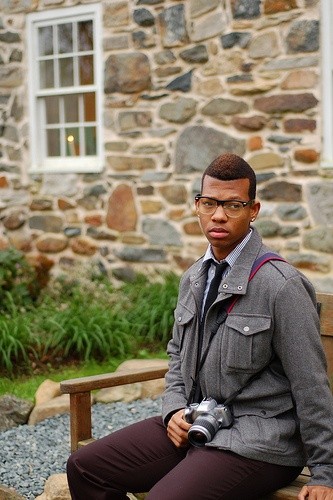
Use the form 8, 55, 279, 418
193, 261, 229, 402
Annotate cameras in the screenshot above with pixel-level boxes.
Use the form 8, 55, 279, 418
183, 396, 233, 449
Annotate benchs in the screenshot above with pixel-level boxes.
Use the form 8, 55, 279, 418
61, 293, 333, 500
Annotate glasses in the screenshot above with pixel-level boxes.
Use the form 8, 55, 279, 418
194, 194, 255, 218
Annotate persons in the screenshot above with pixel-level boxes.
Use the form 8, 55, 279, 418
65, 155, 331, 500
